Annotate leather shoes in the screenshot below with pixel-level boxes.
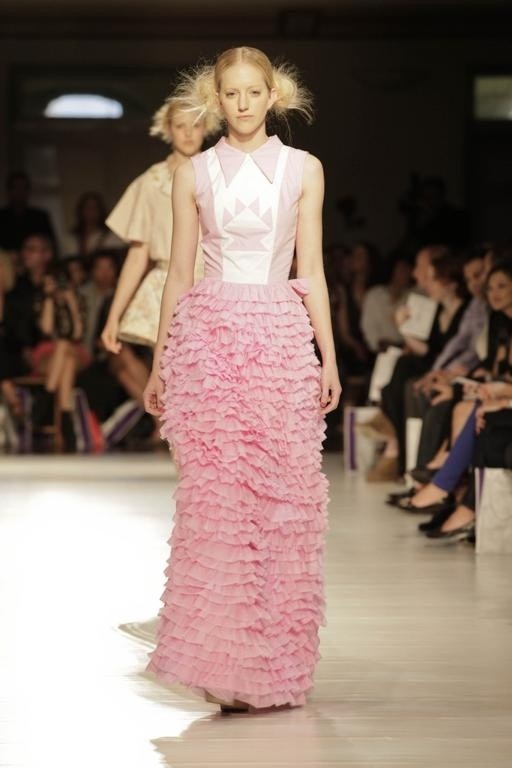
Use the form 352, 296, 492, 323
204, 690, 249, 712
387, 482, 476, 542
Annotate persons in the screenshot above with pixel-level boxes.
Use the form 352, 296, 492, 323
143, 44, 343, 711
100, 100, 209, 354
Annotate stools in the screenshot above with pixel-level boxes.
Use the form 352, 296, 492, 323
343, 406, 511, 552
18, 373, 66, 453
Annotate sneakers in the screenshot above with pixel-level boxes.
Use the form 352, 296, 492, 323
364, 451, 402, 483
354, 412, 400, 441
